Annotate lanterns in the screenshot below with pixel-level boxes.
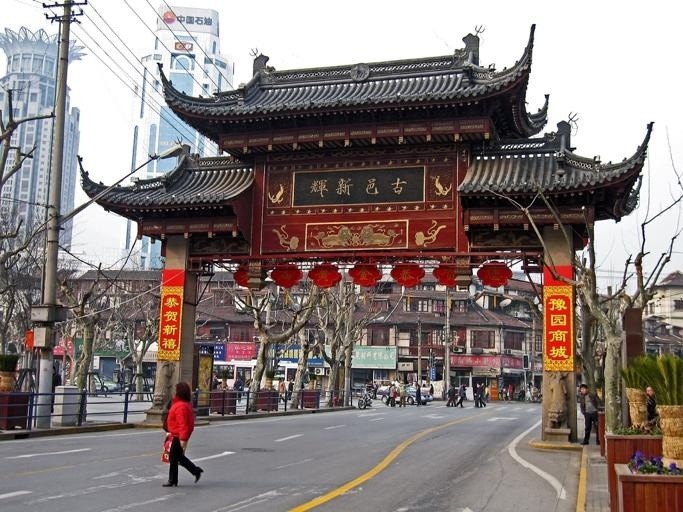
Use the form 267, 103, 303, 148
234, 261, 513, 291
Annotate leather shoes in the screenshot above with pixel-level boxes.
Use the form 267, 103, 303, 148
595, 439, 599, 446
581, 440, 589, 446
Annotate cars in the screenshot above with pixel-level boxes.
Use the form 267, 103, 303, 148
376, 382, 433, 405
66, 372, 121, 393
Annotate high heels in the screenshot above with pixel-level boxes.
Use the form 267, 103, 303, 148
194, 466, 204, 484
162, 479, 178, 488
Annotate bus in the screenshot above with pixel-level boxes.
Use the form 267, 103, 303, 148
211, 360, 310, 393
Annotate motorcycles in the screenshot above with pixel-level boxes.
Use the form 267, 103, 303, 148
358, 392, 372, 409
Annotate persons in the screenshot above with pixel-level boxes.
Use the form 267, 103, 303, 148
212, 371, 245, 404
366, 382, 540, 408
50, 365, 63, 412
162, 382, 204, 486
645, 385, 659, 424
579, 384, 603, 446
277, 377, 294, 403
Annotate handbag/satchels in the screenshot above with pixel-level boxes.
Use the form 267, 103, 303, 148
161, 440, 188, 464
